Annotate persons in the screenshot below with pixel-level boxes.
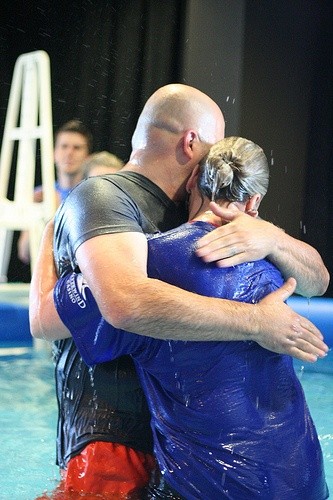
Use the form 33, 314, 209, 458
29, 136, 333, 500
52, 83, 330, 500
16, 118, 91, 266
